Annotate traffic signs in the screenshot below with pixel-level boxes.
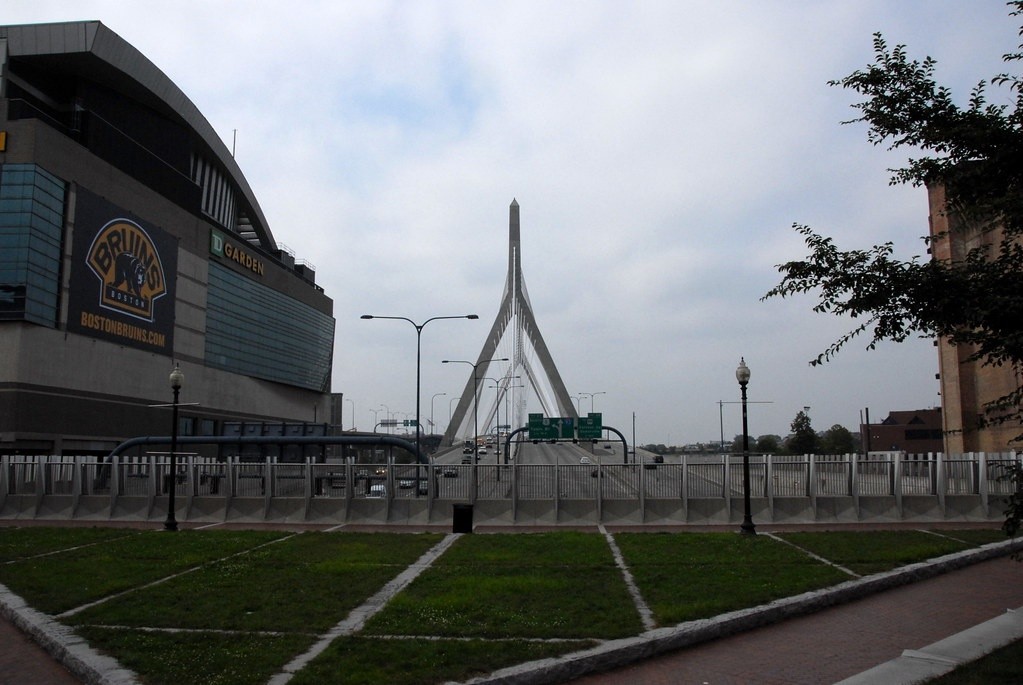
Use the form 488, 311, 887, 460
528, 413, 602, 439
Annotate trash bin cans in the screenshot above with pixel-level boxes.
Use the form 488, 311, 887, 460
451, 502, 474, 534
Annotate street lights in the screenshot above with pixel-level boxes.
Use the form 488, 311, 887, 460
579, 391, 606, 412
489, 385, 524, 437
360, 315, 479, 497
570, 396, 587, 417
450, 397, 461, 421
346, 397, 448, 436
149, 362, 200, 531
442, 358, 510, 465
715, 355, 774, 538
476, 375, 521, 482
431, 393, 447, 434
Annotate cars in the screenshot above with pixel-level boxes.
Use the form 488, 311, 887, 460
627, 446, 633, 453
653, 455, 664, 463
644, 460, 658, 470
461, 431, 507, 464
369, 484, 386, 498
375, 463, 459, 495
580, 457, 591, 464
604, 443, 612, 449
